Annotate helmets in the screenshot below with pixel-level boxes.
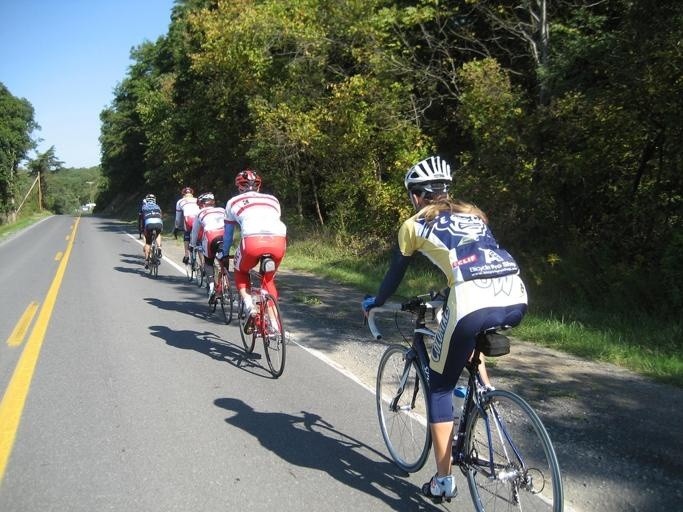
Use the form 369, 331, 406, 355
235, 170, 261, 191
182, 188, 193, 195
145, 194, 156, 203
404, 156, 452, 190
197, 192, 215, 206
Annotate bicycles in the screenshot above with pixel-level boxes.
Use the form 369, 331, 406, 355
139, 230, 160, 278
198, 240, 233, 325
220, 253, 286, 377
363, 291, 565, 511
174, 230, 204, 287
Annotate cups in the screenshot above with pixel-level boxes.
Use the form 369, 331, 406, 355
451, 387, 466, 442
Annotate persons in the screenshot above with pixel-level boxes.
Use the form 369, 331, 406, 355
359, 155, 529, 500
188, 191, 233, 307
213, 170, 287, 341
138, 194, 163, 270
171, 186, 206, 278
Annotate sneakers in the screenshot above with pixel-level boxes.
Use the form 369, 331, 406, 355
145, 265, 148, 269
243, 309, 257, 335
208, 293, 216, 304
423, 474, 458, 499
183, 256, 189, 263
